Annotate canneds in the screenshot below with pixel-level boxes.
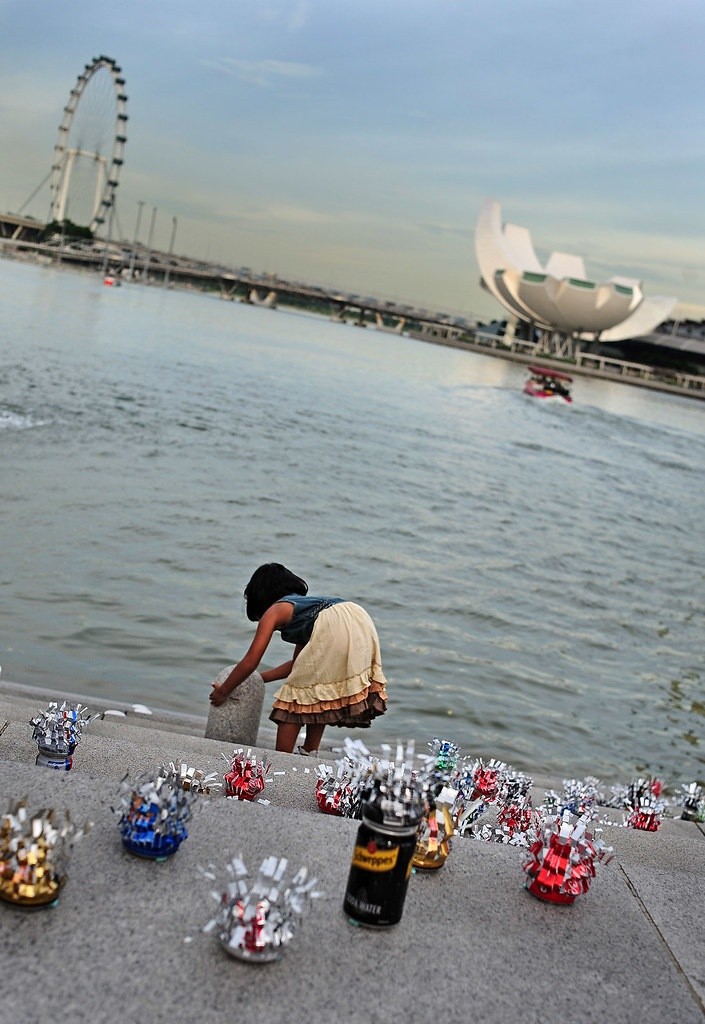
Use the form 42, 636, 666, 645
343, 820, 419, 932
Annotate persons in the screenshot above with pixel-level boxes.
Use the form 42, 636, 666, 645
208, 563, 388, 757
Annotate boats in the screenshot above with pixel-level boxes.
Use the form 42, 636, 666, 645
521, 365, 573, 402
